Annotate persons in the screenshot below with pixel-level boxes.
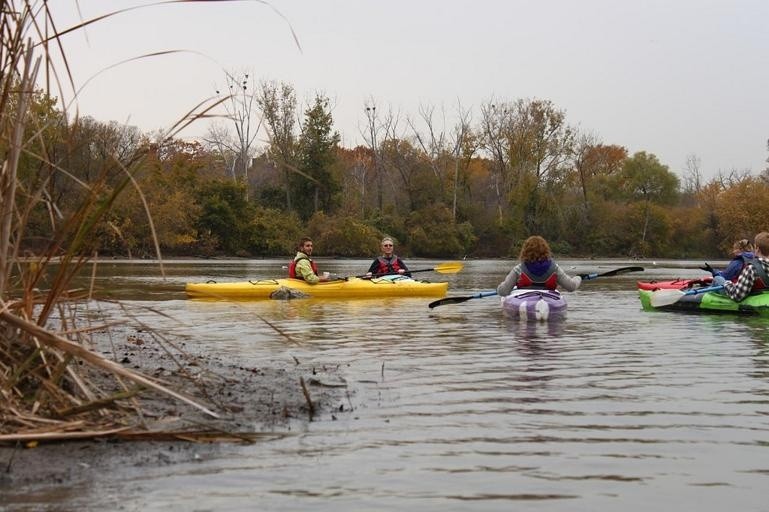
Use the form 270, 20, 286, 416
496, 234, 591, 298
710, 231, 769, 303
288, 236, 335, 284
365, 236, 413, 280
698, 237, 755, 285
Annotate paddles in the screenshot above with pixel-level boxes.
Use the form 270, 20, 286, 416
356, 261, 464, 278
428, 266, 644, 308
650, 283, 729, 307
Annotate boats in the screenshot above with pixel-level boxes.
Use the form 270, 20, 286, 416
185, 276, 449, 300
636, 277, 713, 291
638, 286, 769, 319
500, 288, 567, 323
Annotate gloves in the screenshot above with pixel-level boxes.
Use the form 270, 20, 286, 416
698, 263, 712, 271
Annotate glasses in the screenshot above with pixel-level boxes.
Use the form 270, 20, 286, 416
384, 244, 392, 247
